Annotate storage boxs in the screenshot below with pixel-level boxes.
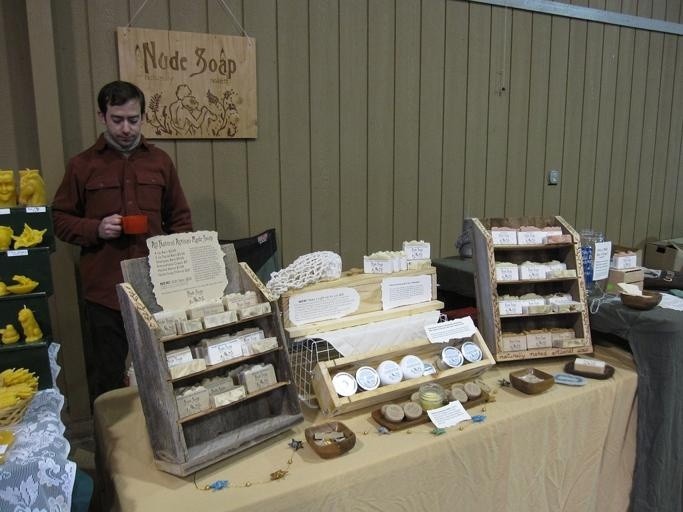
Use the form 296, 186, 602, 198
646, 242, 682, 270
311, 326, 497, 418
606, 255, 645, 295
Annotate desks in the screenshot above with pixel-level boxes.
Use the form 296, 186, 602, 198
431, 258, 683, 512
95, 357, 639, 512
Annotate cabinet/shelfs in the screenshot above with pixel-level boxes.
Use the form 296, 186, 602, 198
1, 206, 55, 390
469, 216, 594, 362
116, 243, 304, 478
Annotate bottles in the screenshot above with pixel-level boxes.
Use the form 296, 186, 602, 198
579, 230, 606, 298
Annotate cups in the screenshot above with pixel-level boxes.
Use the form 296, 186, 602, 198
119, 214, 148, 234
419, 383, 446, 413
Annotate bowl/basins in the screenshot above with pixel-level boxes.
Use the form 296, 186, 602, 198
623, 292, 663, 309
509, 367, 554, 395
305, 422, 356, 459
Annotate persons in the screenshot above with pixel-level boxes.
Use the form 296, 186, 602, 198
51, 80, 192, 512
